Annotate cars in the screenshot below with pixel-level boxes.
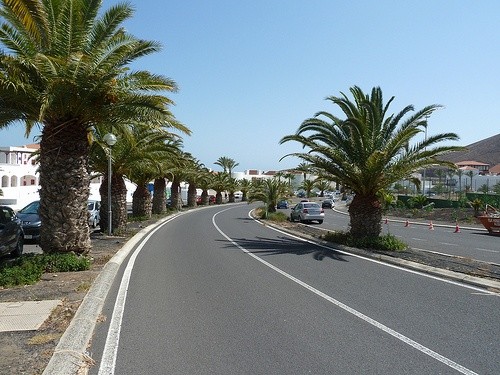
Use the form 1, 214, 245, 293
87, 200, 101, 228
15, 200, 41, 244
166, 190, 188, 208
196, 195, 216, 206
0, 206, 25, 259
289, 201, 325, 224
322, 199, 335, 209
277, 199, 288, 209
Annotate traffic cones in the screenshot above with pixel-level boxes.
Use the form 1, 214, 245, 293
404, 219, 410, 228
428, 221, 435, 230
454, 223, 461, 233
384, 216, 390, 225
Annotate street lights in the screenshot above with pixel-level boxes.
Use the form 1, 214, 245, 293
103, 133, 117, 236
422, 114, 430, 196
